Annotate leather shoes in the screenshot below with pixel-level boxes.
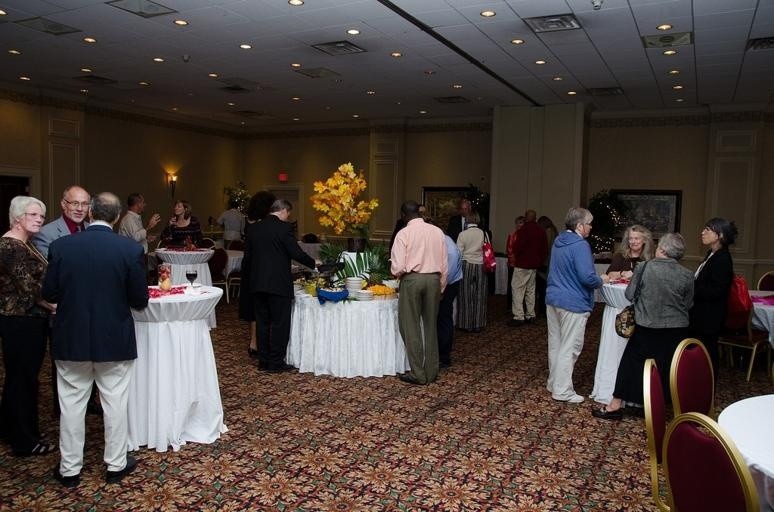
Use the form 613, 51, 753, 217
592, 406, 622, 418
438, 361, 450, 368
258, 364, 294, 372
247, 346, 258, 357
400, 374, 416, 383
106, 455, 137, 483
55, 463, 80, 483
87, 401, 103, 417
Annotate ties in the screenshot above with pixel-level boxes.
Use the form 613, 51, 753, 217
77, 226, 81, 231
464, 218, 466, 230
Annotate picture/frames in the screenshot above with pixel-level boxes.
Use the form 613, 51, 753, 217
609, 190, 683, 243
421, 186, 474, 228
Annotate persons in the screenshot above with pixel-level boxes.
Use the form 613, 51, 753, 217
606, 225, 655, 278
42, 193, 149, 487
689, 218, 738, 398
591, 233, 695, 420
389, 199, 498, 385
161, 200, 204, 250
117, 193, 160, 265
545, 207, 609, 403
208, 190, 324, 374
30, 185, 90, 262
506, 210, 559, 326
1, 196, 57, 457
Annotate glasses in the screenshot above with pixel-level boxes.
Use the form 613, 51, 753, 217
62, 197, 90, 208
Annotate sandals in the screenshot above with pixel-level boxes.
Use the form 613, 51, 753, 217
16, 441, 57, 455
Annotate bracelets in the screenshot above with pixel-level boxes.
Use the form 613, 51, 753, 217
620, 270, 624, 277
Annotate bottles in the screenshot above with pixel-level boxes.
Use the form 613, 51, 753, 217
185, 235, 193, 251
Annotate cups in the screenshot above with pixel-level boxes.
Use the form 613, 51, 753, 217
156, 264, 172, 291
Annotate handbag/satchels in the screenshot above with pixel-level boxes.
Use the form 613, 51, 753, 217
616, 304, 636, 338
728, 274, 753, 315
483, 241, 496, 273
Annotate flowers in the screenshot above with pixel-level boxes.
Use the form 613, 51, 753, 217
311, 163, 379, 232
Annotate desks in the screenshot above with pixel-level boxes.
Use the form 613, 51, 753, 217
718, 394, 774, 511
495, 257, 508, 294
129, 237, 337, 454
590, 280, 637, 407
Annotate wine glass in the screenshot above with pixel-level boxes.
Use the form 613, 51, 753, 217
185, 268, 198, 287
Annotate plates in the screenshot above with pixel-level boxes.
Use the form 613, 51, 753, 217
345, 277, 374, 301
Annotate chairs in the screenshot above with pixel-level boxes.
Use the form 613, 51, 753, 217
643, 269, 774, 512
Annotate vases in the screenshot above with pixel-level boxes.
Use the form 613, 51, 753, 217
346, 239, 364, 251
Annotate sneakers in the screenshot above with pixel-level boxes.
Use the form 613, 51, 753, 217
568, 394, 585, 403
507, 317, 534, 327
